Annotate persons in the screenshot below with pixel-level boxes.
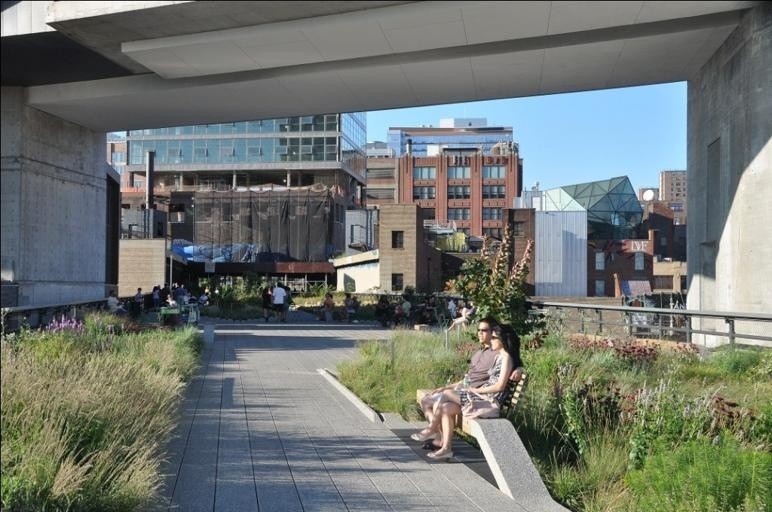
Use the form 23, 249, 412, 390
107, 280, 223, 328
420, 317, 523, 451
259, 282, 304, 323
314, 292, 362, 323
409, 323, 519, 461
374, 293, 462, 328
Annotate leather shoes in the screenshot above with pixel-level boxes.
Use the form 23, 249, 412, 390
410, 432, 454, 461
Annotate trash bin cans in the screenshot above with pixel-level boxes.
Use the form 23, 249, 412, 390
162, 308, 180, 326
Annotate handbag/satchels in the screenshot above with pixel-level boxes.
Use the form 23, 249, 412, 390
461, 389, 501, 419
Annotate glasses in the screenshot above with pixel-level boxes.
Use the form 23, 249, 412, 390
476, 328, 487, 331
491, 336, 498, 339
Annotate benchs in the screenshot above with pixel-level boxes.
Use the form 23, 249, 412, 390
417, 385, 552, 501
